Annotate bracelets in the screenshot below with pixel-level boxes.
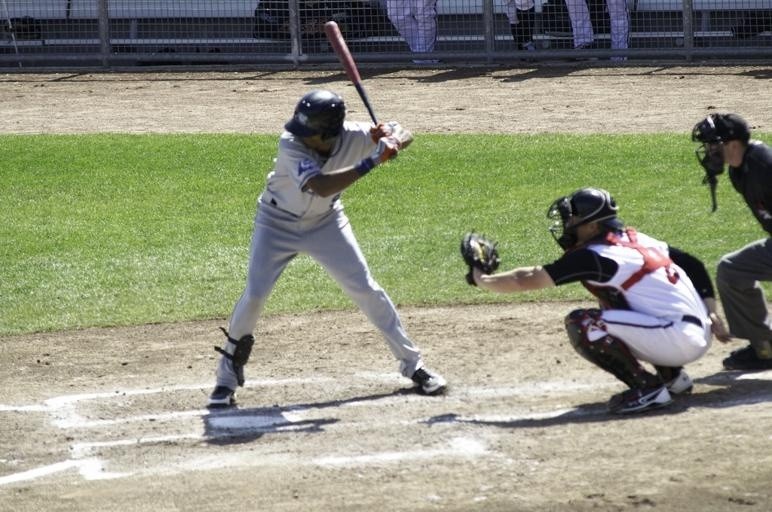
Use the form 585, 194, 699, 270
353, 154, 382, 177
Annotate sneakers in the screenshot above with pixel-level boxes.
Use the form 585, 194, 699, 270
655, 366, 693, 395
206, 386, 236, 405
520, 43, 542, 67
723, 345, 772, 369
412, 366, 447, 393
608, 377, 671, 415
563, 43, 598, 62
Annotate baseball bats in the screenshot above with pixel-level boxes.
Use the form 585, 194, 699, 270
325, 21, 398, 158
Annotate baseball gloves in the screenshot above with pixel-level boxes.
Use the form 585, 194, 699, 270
462, 233, 500, 285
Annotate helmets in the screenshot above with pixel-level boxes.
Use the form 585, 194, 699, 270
692, 113, 750, 210
284, 90, 344, 140
547, 188, 617, 253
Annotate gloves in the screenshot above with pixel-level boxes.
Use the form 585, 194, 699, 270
354, 135, 402, 177
370, 123, 394, 143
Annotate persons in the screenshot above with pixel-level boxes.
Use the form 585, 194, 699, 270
500, 0, 540, 63
203, 91, 445, 409
566, 1, 630, 62
460, 187, 730, 414
691, 113, 772, 373
386, 0, 441, 66
